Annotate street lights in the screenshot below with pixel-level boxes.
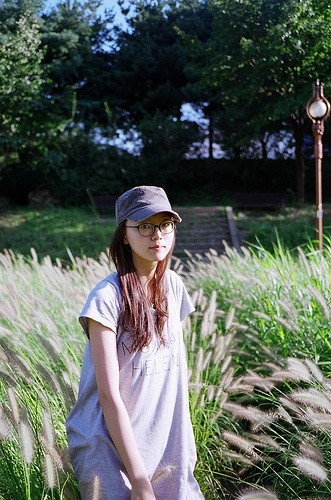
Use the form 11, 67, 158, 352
305, 78, 330, 257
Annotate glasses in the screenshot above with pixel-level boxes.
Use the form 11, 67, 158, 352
122, 219, 175, 236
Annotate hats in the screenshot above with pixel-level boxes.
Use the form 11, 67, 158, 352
115, 186, 182, 225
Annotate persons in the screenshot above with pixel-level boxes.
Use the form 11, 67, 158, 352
64, 185, 207, 500
